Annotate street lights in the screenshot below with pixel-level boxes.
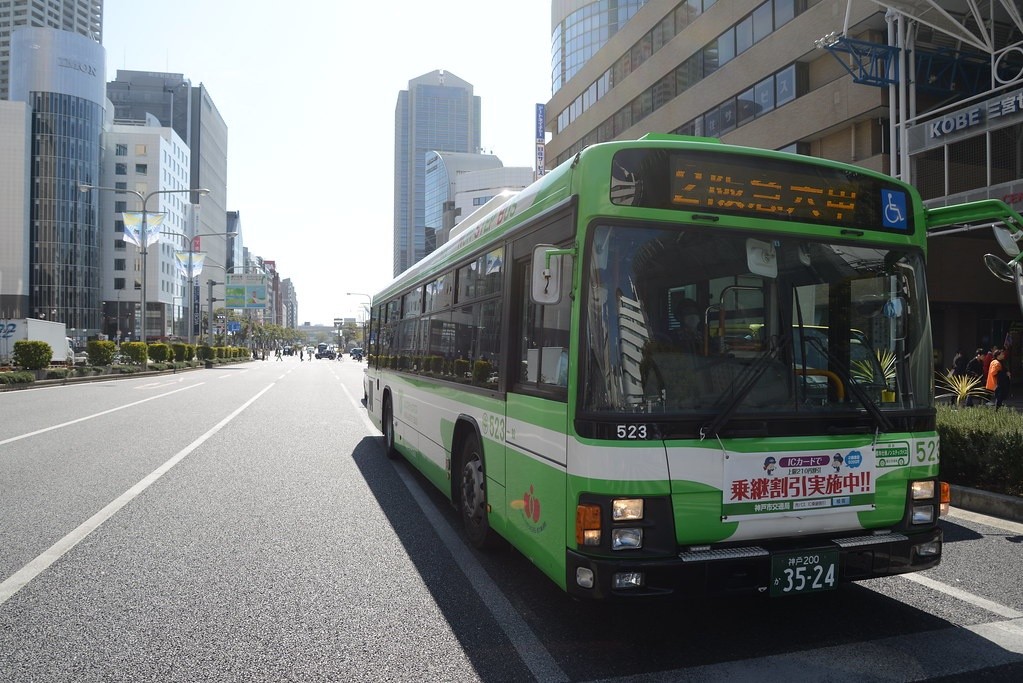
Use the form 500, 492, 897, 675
196, 263, 261, 347
80, 184, 210, 342
347, 292, 372, 326
157, 231, 238, 344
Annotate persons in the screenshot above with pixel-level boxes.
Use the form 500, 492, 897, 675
986, 349, 1010, 411
668, 298, 705, 352
254, 348, 257, 356
307, 350, 311, 361
952, 351, 963, 374
337, 351, 341, 359
983, 345, 1009, 380
300, 350, 304, 361
965, 349, 987, 406
358, 353, 362, 362
276, 350, 283, 361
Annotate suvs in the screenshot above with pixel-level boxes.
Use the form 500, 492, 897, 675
315, 349, 336, 360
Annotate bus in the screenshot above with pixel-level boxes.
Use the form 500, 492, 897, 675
318, 343, 329, 353
360, 131, 1023, 605
709, 324, 886, 408
